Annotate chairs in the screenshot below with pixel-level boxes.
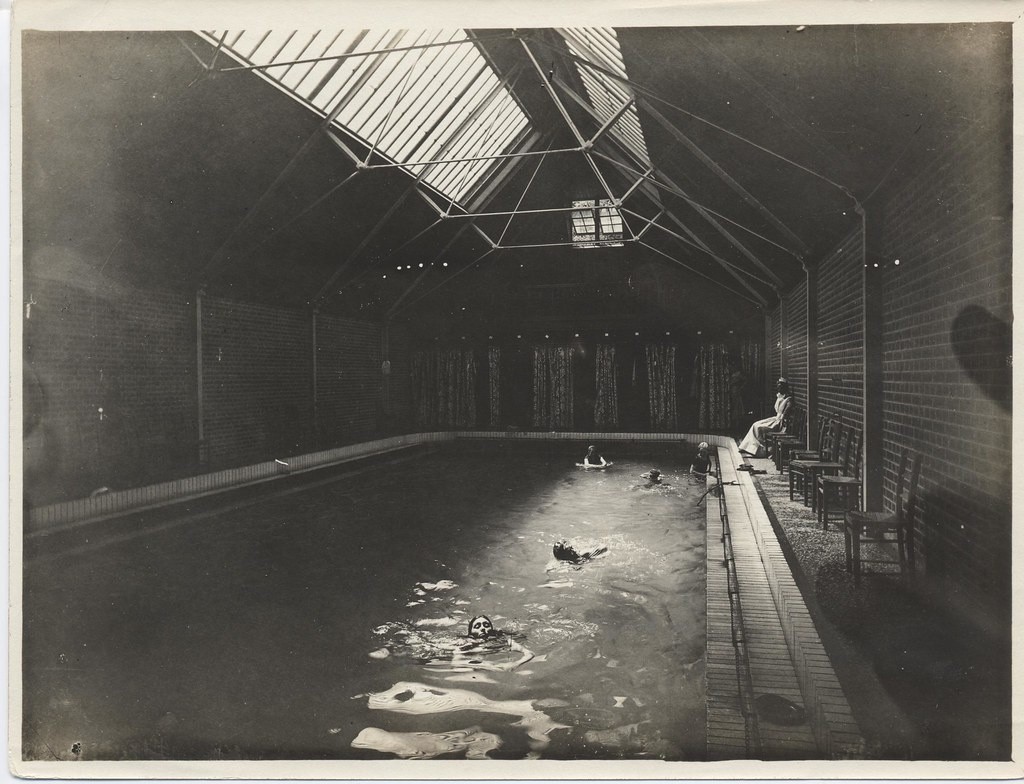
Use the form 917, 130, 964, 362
766, 406, 923, 593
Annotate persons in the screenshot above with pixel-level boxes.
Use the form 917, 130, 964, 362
690, 442, 712, 475
584, 446, 607, 467
469, 614, 492, 636
650, 469, 660, 478
739, 378, 790, 455
554, 538, 577, 558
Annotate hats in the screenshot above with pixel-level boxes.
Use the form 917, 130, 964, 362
698, 441, 710, 450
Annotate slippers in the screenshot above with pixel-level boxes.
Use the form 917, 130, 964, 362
737, 464, 753, 471
